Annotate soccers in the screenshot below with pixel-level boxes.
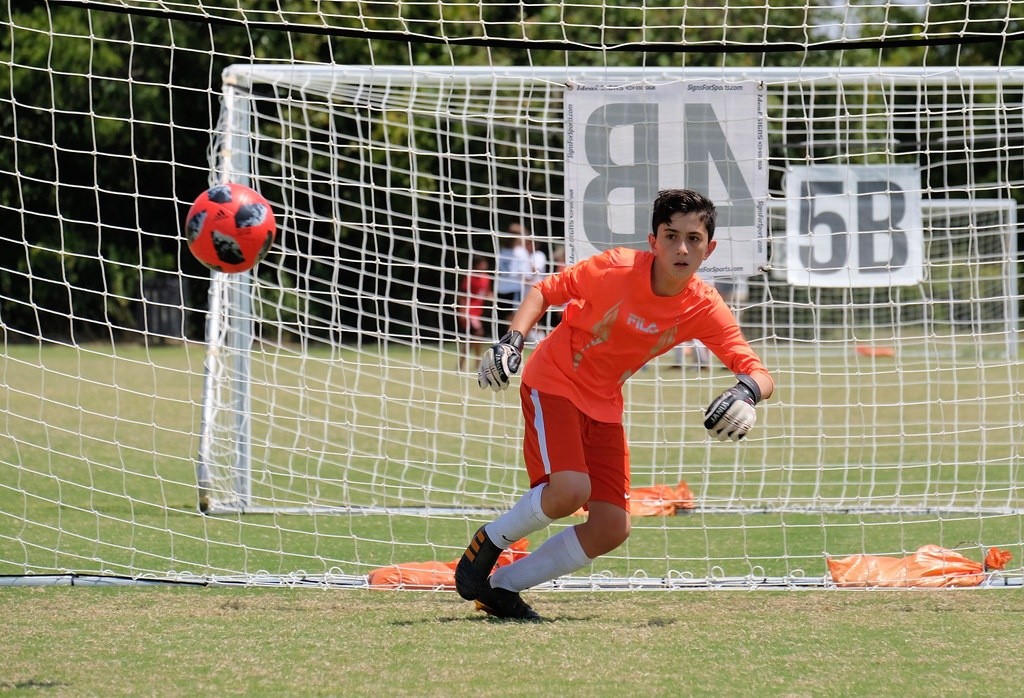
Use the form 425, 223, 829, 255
185, 183, 277, 274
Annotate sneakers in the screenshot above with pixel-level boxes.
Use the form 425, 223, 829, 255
475, 576, 540, 620
455, 524, 504, 601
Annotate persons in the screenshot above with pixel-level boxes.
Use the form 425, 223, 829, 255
455, 188, 775, 621
457, 222, 564, 374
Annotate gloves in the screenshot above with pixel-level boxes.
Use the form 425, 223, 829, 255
477, 330, 524, 392
704, 374, 761, 443
